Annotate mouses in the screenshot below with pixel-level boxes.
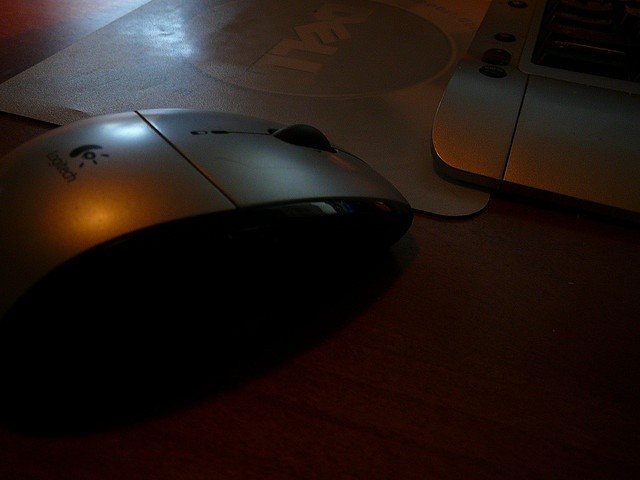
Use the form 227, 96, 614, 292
1, 107, 414, 321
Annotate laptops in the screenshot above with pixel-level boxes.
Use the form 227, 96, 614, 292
430, 1, 639, 228
